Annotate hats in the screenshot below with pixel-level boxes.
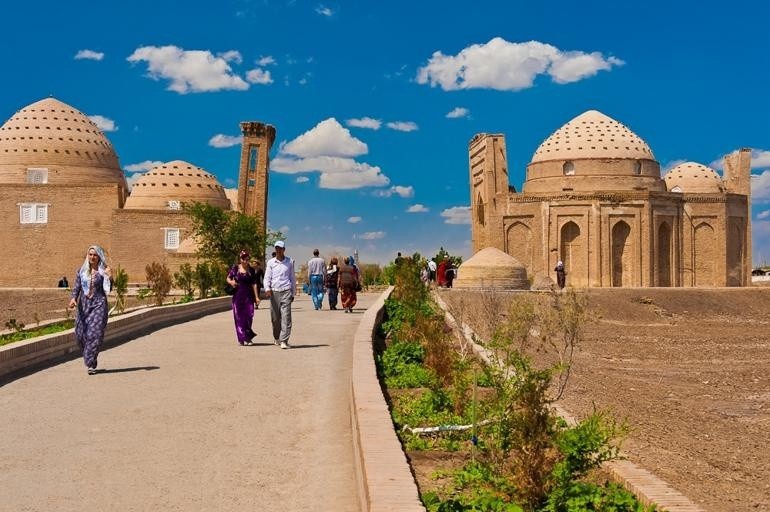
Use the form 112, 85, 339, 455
274, 240, 285, 249
239, 251, 249, 257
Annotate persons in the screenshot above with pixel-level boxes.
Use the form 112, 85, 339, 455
417, 253, 456, 289
251, 259, 265, 309
68, 245, 115, 374
553, 261, 567, 290
271, 252, 276, 258
394, 252, 404, 264
302, 247, 361, 314
225, 250, 261, 346
263, 240, 296, 348
58, 276, 69, 287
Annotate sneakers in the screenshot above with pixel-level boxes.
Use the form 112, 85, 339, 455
274, 339, 280, 346
280, 341, 288, 349
87, 367, 97, 374
245, 340, 253, 345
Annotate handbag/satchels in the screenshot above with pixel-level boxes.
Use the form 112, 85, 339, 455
353, 280, 362, 292
224, 273, 237, 296
